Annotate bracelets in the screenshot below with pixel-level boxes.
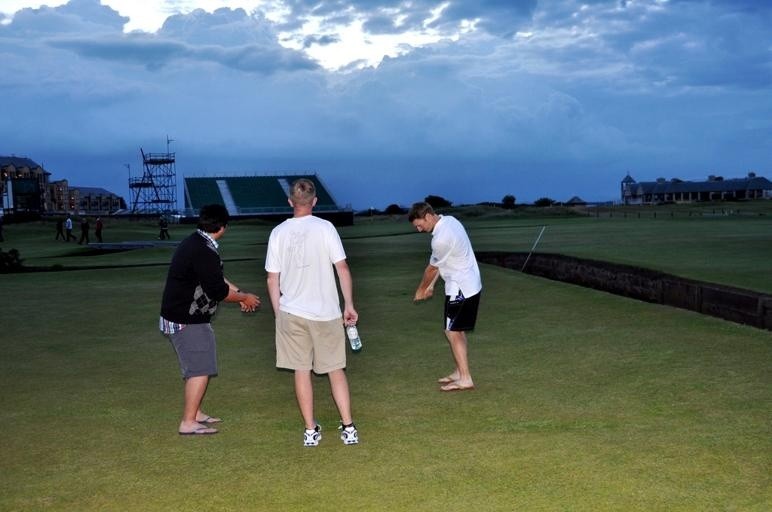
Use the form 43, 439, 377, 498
235, 288, 243, 294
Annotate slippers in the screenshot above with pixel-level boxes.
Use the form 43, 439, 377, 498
437, 374, 476, 392
197, 415, 224, 424
179, 426, 220, 436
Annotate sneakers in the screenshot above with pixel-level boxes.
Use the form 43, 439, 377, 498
340, 422, 360, 445
303, 423, 322, 447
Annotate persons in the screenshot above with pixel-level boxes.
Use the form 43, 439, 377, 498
158, 217, 166, 240
53, 214, 65, 241
161, 215, 171, 239
407, 201, 481, 392
78, 218, 91, 245
260, 177, 364, 445
159, 202, 261, 436
94, 217, 104, 242
65, 214, 78, 241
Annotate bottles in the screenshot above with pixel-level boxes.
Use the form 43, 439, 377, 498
346, 319, 363, 351
